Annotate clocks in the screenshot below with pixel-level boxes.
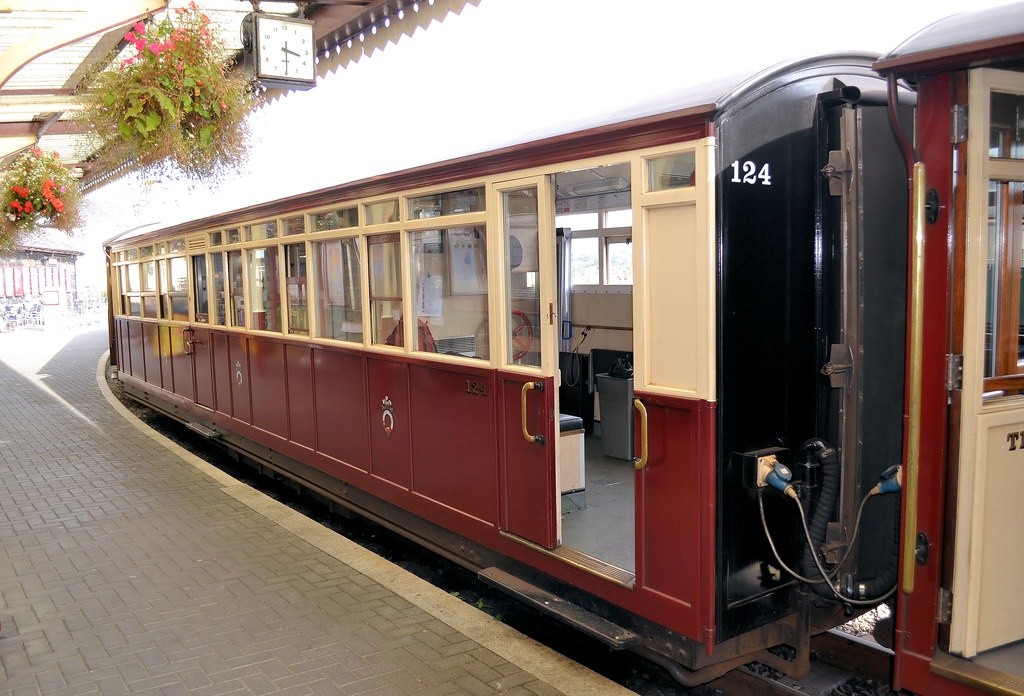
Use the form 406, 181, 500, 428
256, 15, 316, 82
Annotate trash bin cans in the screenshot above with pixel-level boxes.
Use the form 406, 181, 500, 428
594, 372, 635, 461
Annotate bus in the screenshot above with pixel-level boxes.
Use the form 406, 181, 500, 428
102, 2, 1024, 696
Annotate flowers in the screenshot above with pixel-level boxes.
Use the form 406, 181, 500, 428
0, 147, 87, 258
70, 1, 260, 195
